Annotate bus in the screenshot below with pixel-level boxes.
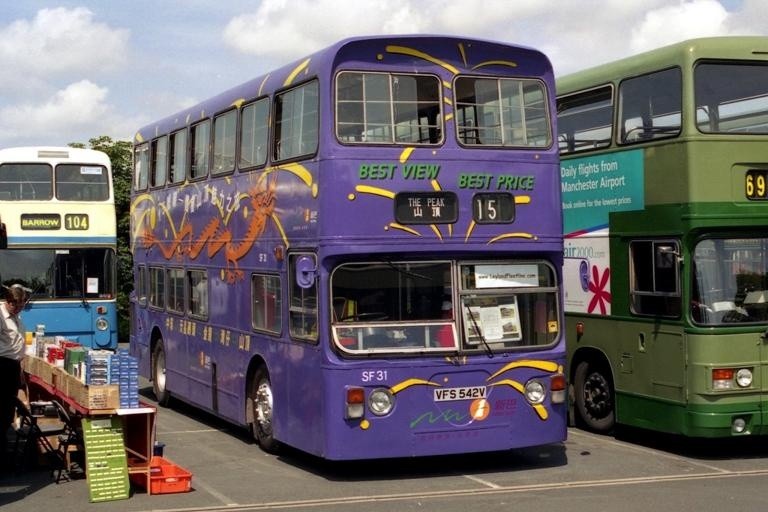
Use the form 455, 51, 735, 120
128, 30, 578, 452
341, 39, 768, 449
0, 145, 118, 359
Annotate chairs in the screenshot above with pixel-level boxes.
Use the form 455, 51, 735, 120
17, 389, 84, 484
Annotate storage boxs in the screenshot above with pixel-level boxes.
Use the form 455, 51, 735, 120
19, 353, 121, 411
129, 441, 193, 495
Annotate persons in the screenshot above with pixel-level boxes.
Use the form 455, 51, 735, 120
0, 286, 27, 462
190, 274, 275, 331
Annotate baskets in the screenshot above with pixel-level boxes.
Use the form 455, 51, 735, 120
125, 455, 192, 494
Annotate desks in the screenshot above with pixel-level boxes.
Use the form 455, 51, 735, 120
27, 373, 157, 498
